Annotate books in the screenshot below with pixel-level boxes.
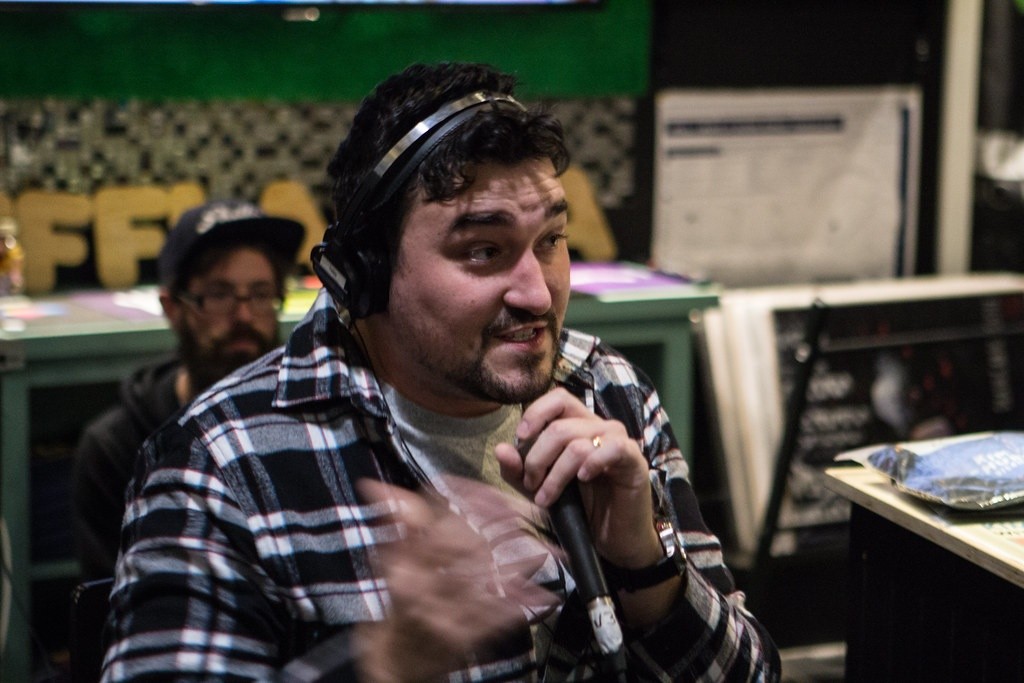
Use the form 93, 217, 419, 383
557, 258, 716, 305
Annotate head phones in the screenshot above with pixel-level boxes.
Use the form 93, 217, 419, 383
308, 90, 566, 316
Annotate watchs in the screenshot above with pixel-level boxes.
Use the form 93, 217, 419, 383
602, 519, 690, 595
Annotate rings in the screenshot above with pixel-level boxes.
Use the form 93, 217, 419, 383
588, 432, 606, 455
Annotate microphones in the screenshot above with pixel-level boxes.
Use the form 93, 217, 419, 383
516, 417, 633, 678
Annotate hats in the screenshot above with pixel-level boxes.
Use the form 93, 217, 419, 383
157, 203, 304, 287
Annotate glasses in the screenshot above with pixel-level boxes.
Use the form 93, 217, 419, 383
170, 278, 290, 318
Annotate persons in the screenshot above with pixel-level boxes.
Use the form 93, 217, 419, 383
63, 194, 315, 658
77, 56, 789, 683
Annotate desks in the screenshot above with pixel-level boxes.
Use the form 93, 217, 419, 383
0, 257, 721, 682
821, 466, 1024, 682
